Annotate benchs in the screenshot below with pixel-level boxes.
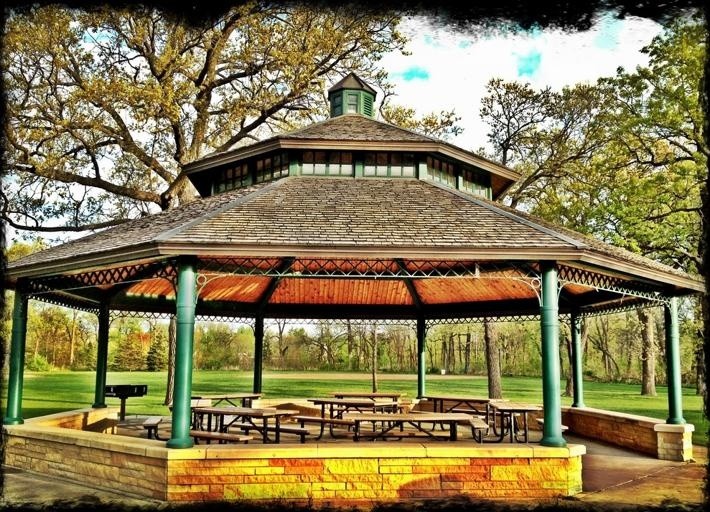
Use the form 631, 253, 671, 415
536, 419, 568, 433
141, 417, 169, 442
